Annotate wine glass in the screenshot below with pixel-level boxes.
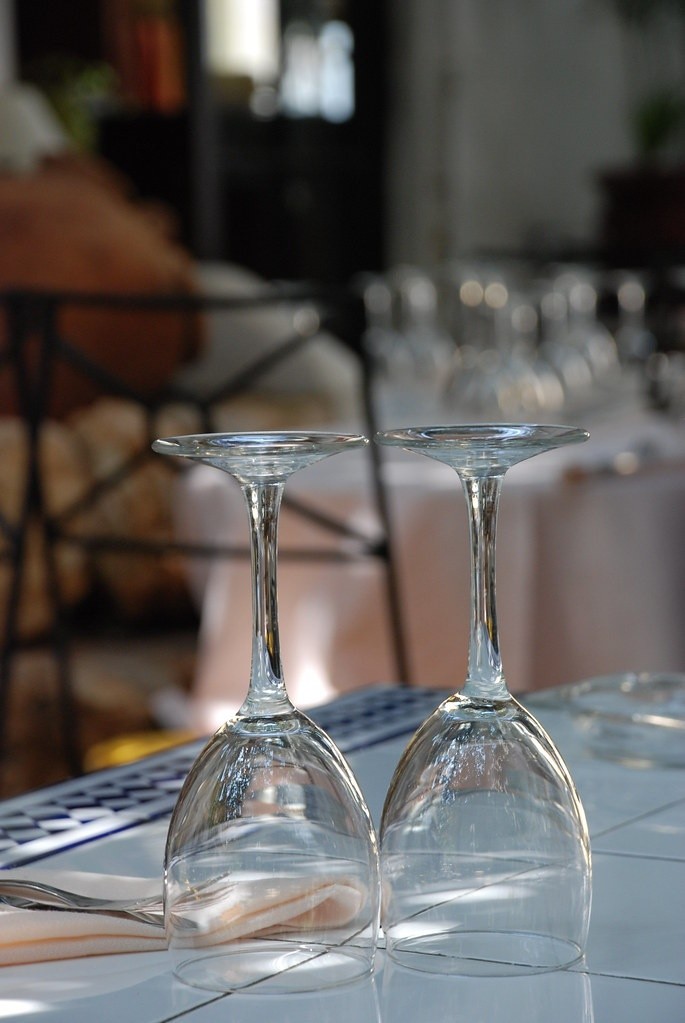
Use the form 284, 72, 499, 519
153, 436, 382, 994
350, 260, 653, 443
376, 425, 598, 977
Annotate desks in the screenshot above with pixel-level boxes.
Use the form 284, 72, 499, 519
0, 675, 685, 1023
176, 401, 685, 718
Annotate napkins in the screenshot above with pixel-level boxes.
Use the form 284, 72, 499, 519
0, 865, 368, 970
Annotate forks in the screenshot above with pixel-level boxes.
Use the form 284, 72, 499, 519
0, 875, 238, 913
